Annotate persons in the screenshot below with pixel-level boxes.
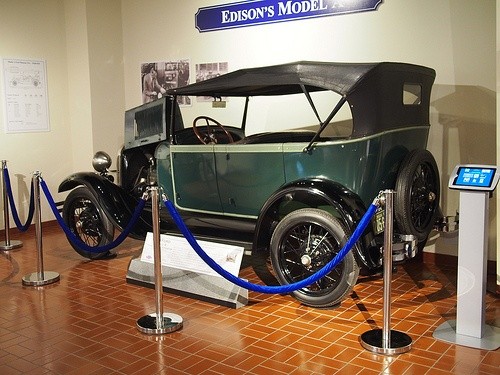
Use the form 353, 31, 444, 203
178, 62, 189, 87
142, 67, 166, 104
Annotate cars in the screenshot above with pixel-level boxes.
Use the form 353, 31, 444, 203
58, 60, 443, 310
